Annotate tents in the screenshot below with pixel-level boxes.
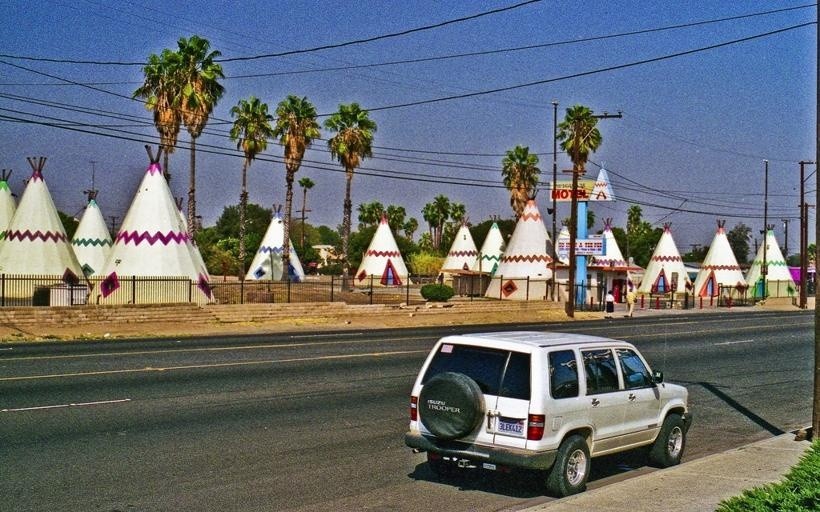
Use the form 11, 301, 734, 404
0, 154, 93, 300
436, 188, 636, 298
638, 218, 800, 298
0, 168, 17, 271
88, 144, 218, 305
245, 204, 308, 282
351, 209, 417, 286
69, 188, 115, 276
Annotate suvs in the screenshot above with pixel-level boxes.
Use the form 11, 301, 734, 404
399, 330, 696, 499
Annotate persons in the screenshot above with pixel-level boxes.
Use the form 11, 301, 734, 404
603, 289, 616, 319
624, 288, 638, 318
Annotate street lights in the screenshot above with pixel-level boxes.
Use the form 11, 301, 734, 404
759, 156, 771, 303
549, 95, 561, 302
